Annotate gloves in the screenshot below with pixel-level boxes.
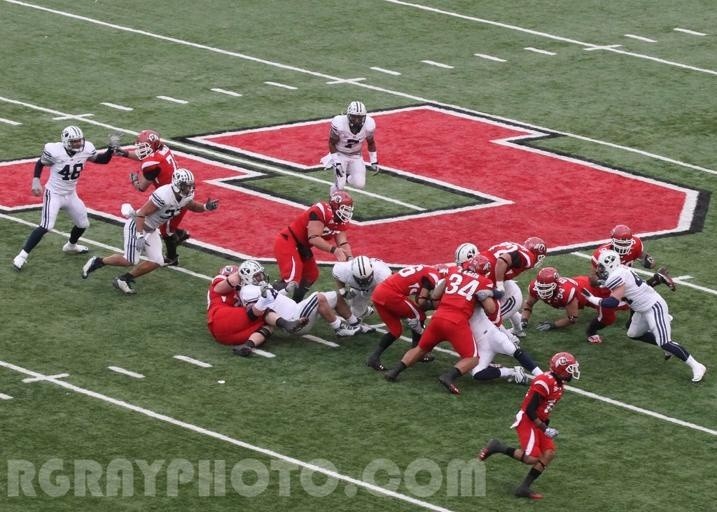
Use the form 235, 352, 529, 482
111, 147, 129, 158
371, 162, 379, 176
536, 319, 557, 333
130, 170, 139, 185
254, 281, 277, 313
109, 135, 120, 147
135, 232, 150, 256
336, 163, 344, 177
539, 422, 558, 438
580, 288, 602, 307
204, 197, 219, 211
32, 177, 43, 196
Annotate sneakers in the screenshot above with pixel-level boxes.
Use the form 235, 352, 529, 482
82, 255, 102, 279
438, 374, 461, 395
549, 352, 581, 381
232, 342, 252, 357
664, 340, 678, 360
176, 231, 190, 246
514, 488, 544, 499
63, 243, 88, 252
367, 354, 397, 381
690, 362, 706, 382
480, 439, 499, 460
286, 317, 310, 334
506, 327, 525, 345
655, 267, 676, 292
334, 305, 376, 337
586, 326, 601, 343
113, 277, 137, 294
512, 366, 535, 385
418, 352, 436, 362
163, 253, 179, 267
13, 255, 27, 270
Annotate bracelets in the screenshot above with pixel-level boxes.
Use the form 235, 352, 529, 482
203, 202, 208, 211
330, 152, 341, 164
136, 231, 144, 239
368, 150, 377, 164
537, 423, 548, 432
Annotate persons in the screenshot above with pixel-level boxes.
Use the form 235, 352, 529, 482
477, 351, 583, 503
111, 129, 193, 269
80, 166, 219, 293
204, 191, 709, 398
327, 98, 380, 198
11, 125, 117, 273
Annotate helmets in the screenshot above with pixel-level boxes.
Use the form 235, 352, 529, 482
594, 250, 622, 281
433, 243, 490, 278
348, 101, 366, 131
60, 126, 84, 152
537, 264, 559, 300
609, 225, 633, 256
134, 130, 160, 161
351, 258, 374, 289
330, 191, 354, 224
219, 259, 267, 285
524, 237, 548, 268
172, 168, 195, 198
